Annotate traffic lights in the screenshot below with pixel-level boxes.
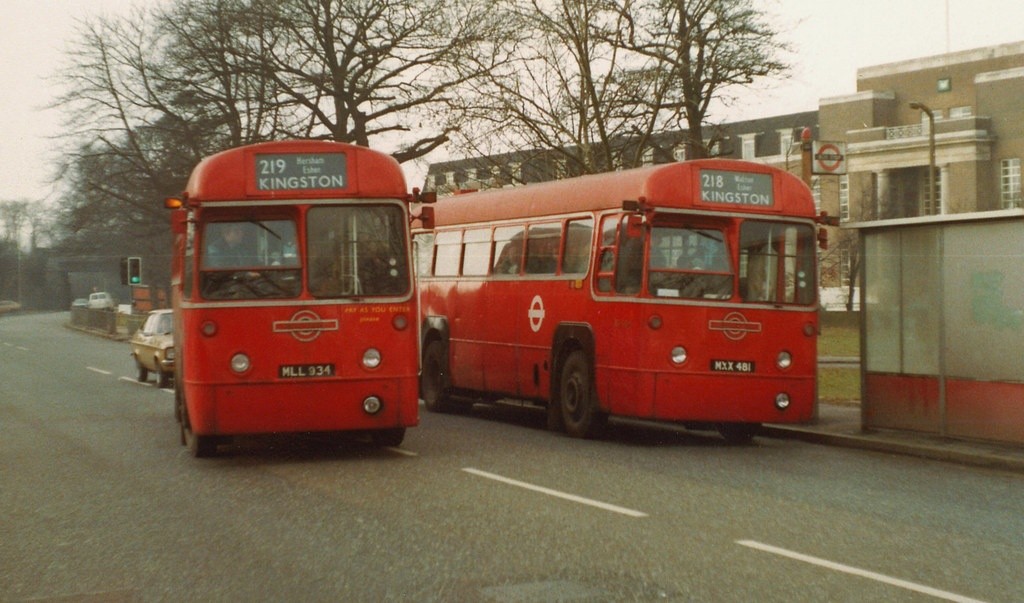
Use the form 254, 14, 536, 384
127, 257, 141, 286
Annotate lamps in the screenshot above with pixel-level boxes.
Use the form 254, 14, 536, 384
935, 77, 953, 93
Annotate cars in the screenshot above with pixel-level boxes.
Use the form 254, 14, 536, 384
127, 309, 175, 388
70, 298, 88, 309
0, 299, 21, 313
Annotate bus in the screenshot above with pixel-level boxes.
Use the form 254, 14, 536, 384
165, 139, 433, 455
412, 157, 829, 442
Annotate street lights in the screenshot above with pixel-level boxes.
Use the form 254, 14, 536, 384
908, 102, 936, 217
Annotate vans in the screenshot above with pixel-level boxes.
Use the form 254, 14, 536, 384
88, 293, 115, 311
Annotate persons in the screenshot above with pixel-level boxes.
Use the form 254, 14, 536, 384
354, 233, 389, 285
532, 240, 568, 274
496, 245, 522, 274
202, 221, 264, 279
